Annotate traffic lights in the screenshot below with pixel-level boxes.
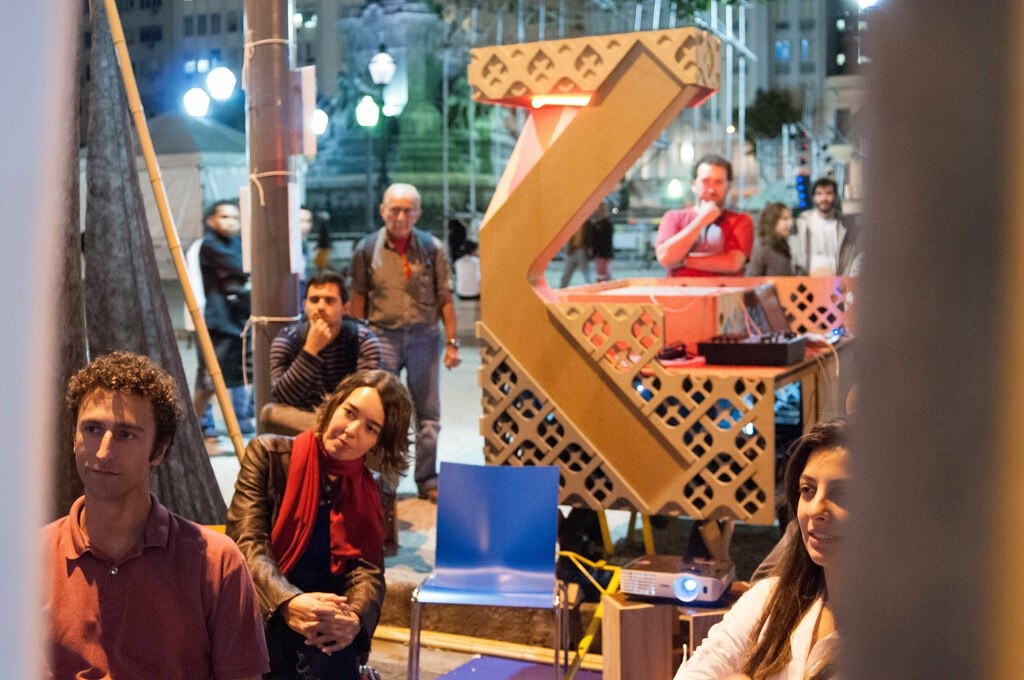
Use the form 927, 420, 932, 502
826, 0, 859, 75
796, 175, 813, 209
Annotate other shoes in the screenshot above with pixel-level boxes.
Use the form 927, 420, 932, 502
419, 486, 437, 504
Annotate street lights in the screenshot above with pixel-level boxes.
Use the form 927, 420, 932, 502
356, 41, 414, 229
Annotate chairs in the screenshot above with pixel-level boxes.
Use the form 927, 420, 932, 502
408, 465, 572, 680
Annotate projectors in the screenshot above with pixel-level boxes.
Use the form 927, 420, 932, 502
620, 553, 735, 603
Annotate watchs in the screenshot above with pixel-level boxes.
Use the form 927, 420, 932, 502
446, 337, 460, 348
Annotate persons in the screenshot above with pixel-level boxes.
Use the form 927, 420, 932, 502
592, 201, 615, 283
560, 219, 593, 288
314, 220, 337, 272
673, 414, 873, 680
225, 370, 412, 680
258, 275, 387, 473
749, 203, 794, 276
788, 178, 847, 276
655, 155, 754, 278
183, 209, 257, 440
33, 352, 270, 680
247, 205, 315, 417
350, 183, 461, 505
192, 202, 252, 418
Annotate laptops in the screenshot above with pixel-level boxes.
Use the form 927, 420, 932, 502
756, 285, 841, 344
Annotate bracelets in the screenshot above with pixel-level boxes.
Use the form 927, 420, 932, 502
679, 259, 685, 268
